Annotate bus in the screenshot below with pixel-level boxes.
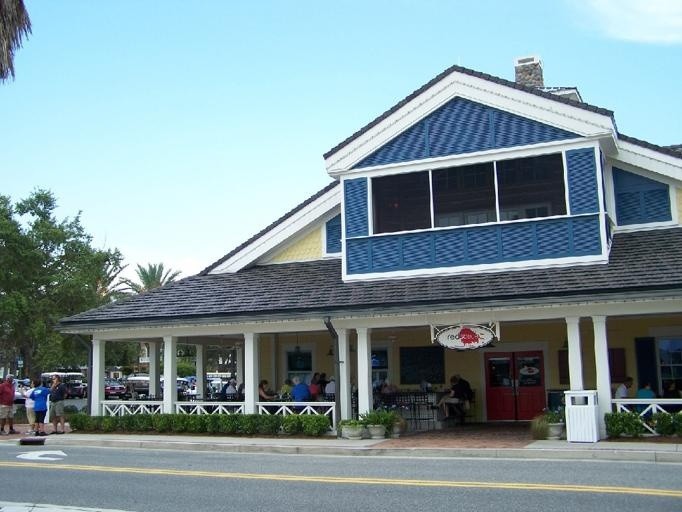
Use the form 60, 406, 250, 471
41, 372, 83, 398
128, 377, 188, 394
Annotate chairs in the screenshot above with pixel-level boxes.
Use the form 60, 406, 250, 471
210, 390, 477, 429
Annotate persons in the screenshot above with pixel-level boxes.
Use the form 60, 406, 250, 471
635, 382, 657, 417
160, 371, 357, 414
0, 376, 55, 400
48, 375, 67, 434
431, 374, 473, 421
125, 381, 134, 400
373, 375, 397, 408
107, 375, 127, 384
0, 373, 20, 435
658, 380, 682, 413
62, 377, 67, 383
30, 379, 50, 436
20, 379, 40, 435
614, 377, 634, 413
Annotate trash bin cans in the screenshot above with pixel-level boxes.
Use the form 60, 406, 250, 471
563, 389, 600, 442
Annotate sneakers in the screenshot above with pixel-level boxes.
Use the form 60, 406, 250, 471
26, 430, 65, 436
1, 427, 21, 434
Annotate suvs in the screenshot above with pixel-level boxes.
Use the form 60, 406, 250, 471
64, 384, 83, 398
105, 378, 127, 399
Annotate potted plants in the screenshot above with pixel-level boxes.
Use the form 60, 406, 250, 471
339, 409, 406, 440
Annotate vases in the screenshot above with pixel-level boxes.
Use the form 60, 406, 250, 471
546, 423, 565, 440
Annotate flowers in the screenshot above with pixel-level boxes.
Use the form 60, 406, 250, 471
532, 406, 565, 435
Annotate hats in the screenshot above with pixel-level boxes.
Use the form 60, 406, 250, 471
329, 376, 336, 382
293, 377, 302, 385
229, 378, 237, 384
